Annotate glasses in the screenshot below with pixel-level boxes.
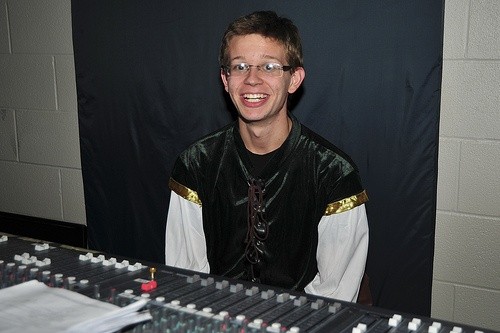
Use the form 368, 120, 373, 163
221, 62, 300, 76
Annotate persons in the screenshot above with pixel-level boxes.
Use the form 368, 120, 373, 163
165, 9, 369, 305
